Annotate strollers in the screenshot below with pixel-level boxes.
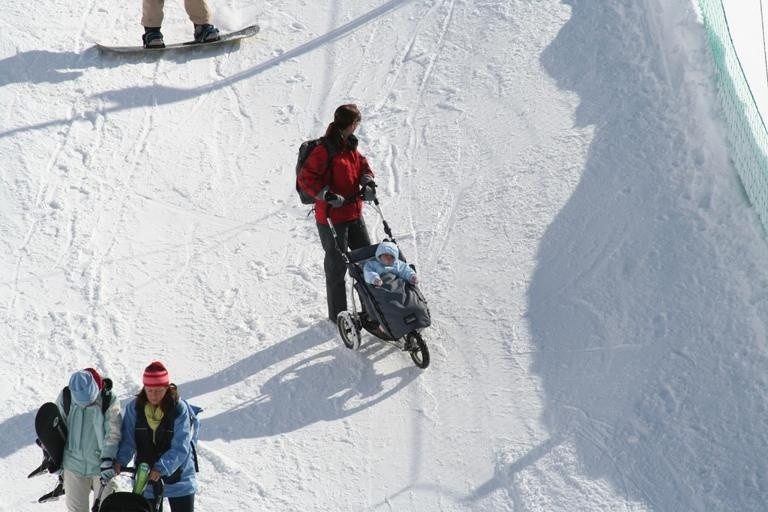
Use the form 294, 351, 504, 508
322, 191, 436, 373
87, 465, 168, 512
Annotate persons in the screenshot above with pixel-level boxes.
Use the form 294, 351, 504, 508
143, 0, 220, 49
296, 102, 377, 326
364, 241, 430, 337
25, 366, 124, 512
114, 360, 206, 512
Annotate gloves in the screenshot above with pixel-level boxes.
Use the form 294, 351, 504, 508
364, 185, 375, 200
326, 192, 344, 208
100, 458, 114, 480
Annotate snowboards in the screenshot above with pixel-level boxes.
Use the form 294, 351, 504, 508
35, 402, 68, 465
96, 25, 260, 53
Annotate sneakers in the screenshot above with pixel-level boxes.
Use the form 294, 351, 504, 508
194, 24, 218, 40
145, 27, 163, 46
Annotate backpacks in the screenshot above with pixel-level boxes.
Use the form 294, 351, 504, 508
296, 137, 333, 204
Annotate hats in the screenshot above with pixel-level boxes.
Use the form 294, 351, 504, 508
69, 371, 99, 405
143, 362, 169, 387
375, 241, 399, 264
